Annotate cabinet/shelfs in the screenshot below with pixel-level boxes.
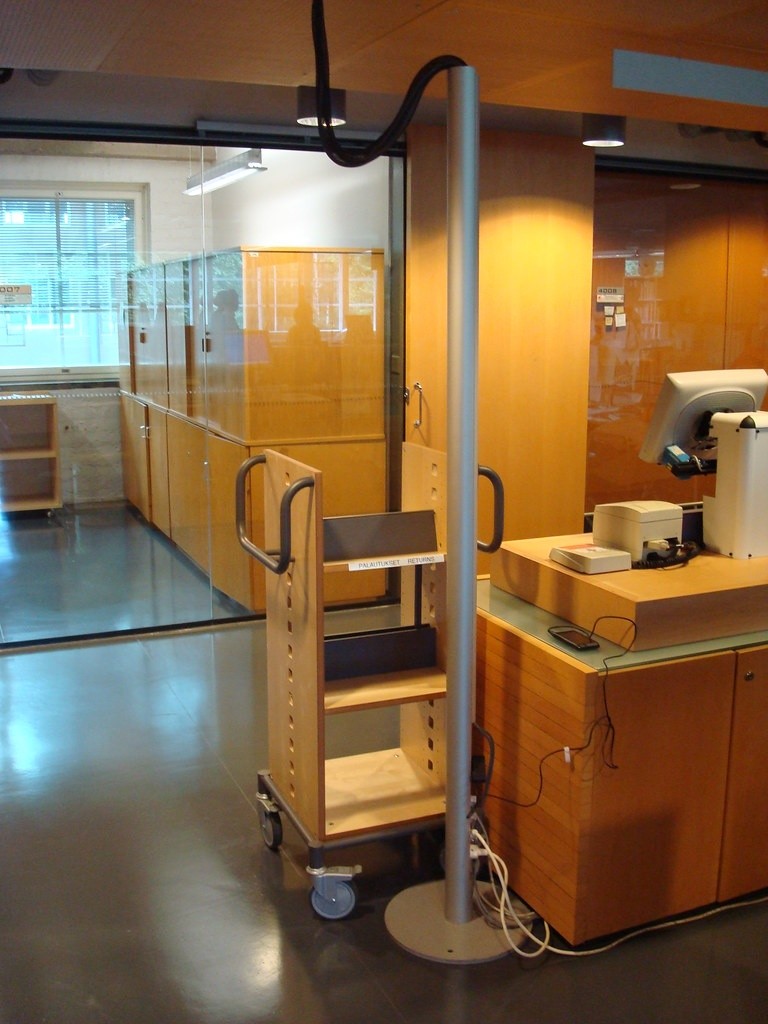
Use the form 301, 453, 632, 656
229, 441, 507, 921
473, 573, 768, 948
114, 263, 170, 410
117, 390, 171, 547
166, 413, 392, 614
0, 390, 62, 518
163, 245, 386, 446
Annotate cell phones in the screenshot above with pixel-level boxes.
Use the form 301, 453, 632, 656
553, 630, 600, 651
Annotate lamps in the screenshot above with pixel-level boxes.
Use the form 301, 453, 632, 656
580, 113, 627, 149
296, 85, 348, 127
182, 146, 268, 197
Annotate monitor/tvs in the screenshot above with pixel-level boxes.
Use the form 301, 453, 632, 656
639, 369, 768, 463
